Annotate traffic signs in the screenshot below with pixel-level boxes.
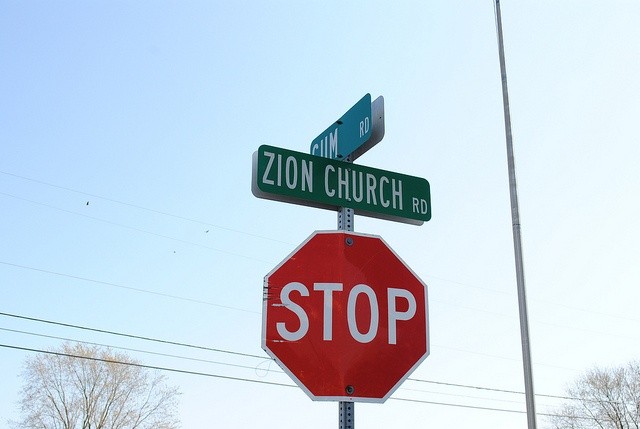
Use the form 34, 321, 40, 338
309, 93, 372, 161
253, 144, 432, 227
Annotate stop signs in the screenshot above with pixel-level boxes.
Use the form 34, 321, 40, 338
261, 230, 430, 403
